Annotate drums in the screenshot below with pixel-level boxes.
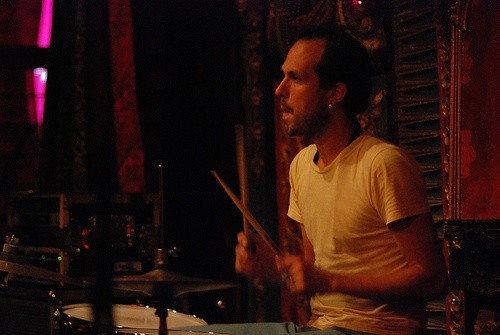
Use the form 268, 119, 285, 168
51, 302, 230, 335
0, 256, 100, 304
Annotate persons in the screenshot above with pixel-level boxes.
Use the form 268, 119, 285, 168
179, 22, 449, 334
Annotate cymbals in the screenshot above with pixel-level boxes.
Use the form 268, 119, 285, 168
107, 268, 235, 299
